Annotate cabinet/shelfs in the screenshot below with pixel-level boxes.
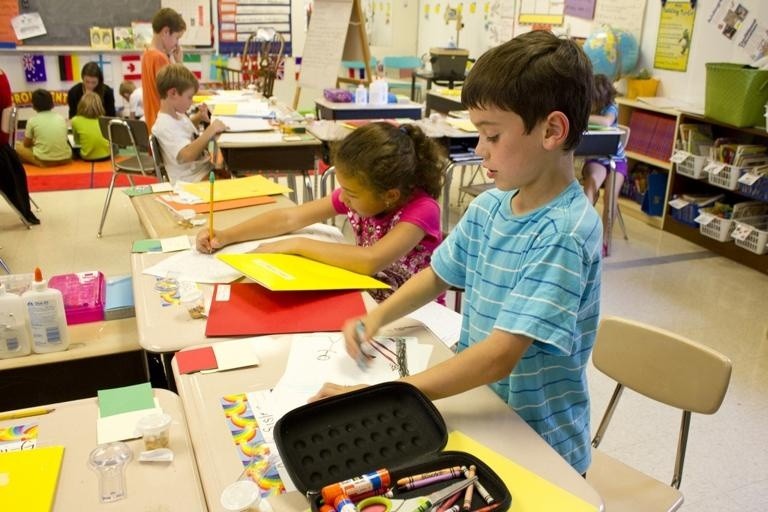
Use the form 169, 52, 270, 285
615, 97, 677, 241
664, 108, 766, 277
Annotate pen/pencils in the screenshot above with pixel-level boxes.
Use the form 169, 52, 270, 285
350, 465, 494, 512
355, 320, 367, 375
209, 172, 215, 254
0, 408, 56, 421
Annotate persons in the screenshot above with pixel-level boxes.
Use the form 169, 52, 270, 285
581, 74, 628, 258
120, 81, 145, 121
67, 62, 115, 117
142, 7, 187, 134
68, 91, 119, 162
150, 63, 231, 184
196, 122, 446, 306
15, 89, 73, 167
307, 29, 604, 480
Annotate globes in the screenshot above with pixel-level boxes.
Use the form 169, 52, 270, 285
583, 21, 640, 81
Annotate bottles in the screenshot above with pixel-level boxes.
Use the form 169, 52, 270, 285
0, 284, 30, 358
354, 77, 388, 106
136, 413, 172, 450
220, 480, 262, 512
23, 268, 71, 352
447, 36, 456, 49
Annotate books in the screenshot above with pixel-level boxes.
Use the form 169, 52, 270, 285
672, 123, 768, 222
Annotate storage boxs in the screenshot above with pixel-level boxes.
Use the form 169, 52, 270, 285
703, 59, 765, 129
429, 46, 470, 79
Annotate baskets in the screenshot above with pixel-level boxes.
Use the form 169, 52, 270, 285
674, 148, 768, 202
671, 194, 768, 256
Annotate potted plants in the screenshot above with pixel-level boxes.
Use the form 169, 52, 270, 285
626, 68, 659, 100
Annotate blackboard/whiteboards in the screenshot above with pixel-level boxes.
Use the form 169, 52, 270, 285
0, 0, 216, 52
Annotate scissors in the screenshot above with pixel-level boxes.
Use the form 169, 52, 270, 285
355, 475, 478, 512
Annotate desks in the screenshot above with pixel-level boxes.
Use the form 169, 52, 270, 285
196, 90, 322, 205
302, 77, 496, 234
0, 313, 153, 386
0, 386, 208, 512
574, 123, 625, 257
168, 313, 604, 512
128, 225, 379, 377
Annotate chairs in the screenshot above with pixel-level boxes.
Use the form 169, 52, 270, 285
580, 125, 630, 240
95, 109, 152, 238
434, 229, 467, 318
1, 103, 42, 230
582, 312, 732, 512
343, 54, 424, 102
144, 127, 168, 187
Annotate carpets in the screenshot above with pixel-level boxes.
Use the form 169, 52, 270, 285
19, 153, 161, 194
